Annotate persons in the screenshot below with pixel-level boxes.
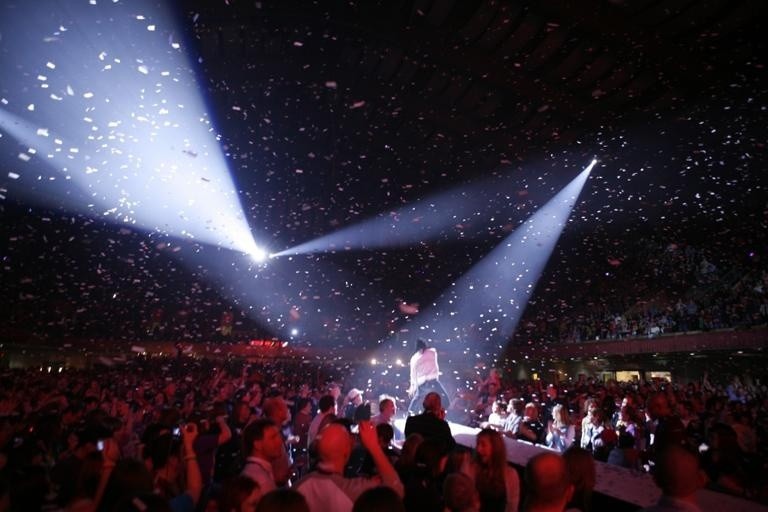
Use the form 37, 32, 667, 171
1, 232, 768, 511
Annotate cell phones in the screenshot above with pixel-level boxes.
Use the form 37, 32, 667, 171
96, 439, 105, 452
349, 423, 360, 436
170, 426, 181, 440
